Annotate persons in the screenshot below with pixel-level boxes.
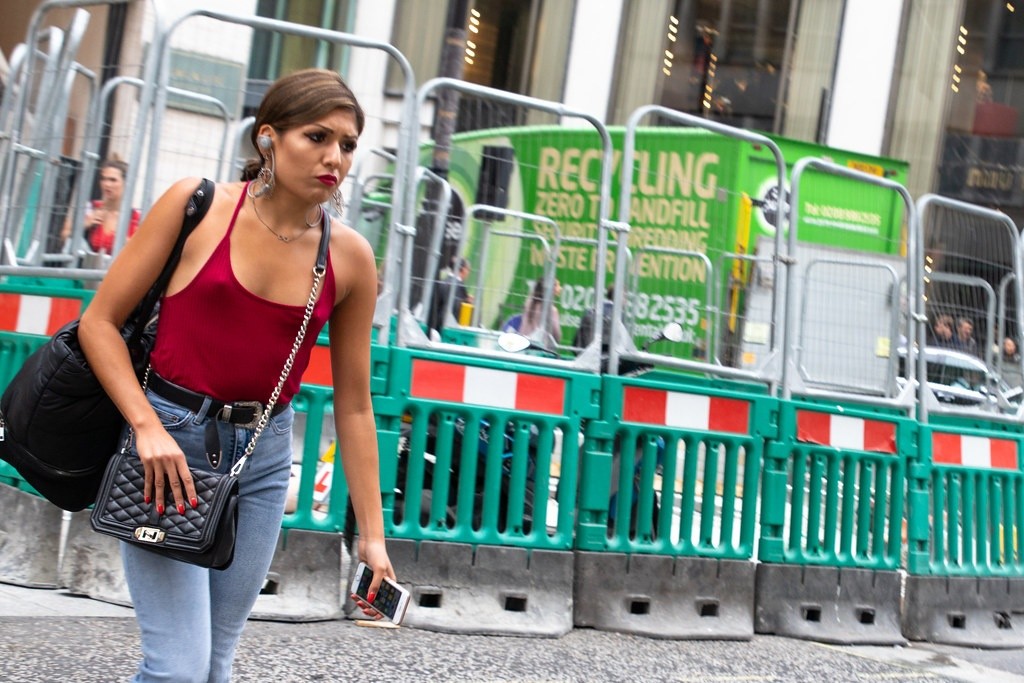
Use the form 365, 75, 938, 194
375, 257, 642, 374
78, 68, 395, 683
898, 315, 1023, 408
62, 159, 141, 267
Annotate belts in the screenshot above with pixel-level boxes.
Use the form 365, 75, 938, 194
146, 371, 291, 424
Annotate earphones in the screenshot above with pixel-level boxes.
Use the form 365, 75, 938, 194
258, 137, 273, 151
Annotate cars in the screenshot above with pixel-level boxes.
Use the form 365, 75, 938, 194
895, 346, 1023, 416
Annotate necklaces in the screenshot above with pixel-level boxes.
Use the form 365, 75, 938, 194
251, 181, 320, 243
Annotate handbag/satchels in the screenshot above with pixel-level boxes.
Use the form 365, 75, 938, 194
90, 444, 242, 572
7, 317, 151, 507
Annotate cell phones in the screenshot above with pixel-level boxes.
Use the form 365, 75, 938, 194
351, 562, 410, 625
